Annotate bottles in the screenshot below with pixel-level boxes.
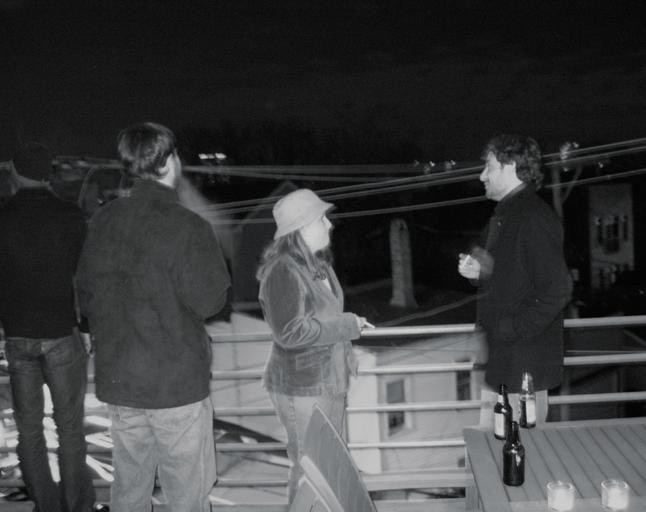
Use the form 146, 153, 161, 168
501, 422, 525, 486
518, 373, 537, 428
492, 384, 513, 438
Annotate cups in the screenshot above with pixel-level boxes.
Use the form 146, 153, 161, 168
546, 482, 576, 512
599, 477, 628, 512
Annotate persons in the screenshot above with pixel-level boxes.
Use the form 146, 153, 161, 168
457, 132, 576, 429
1, 137, 110, 510
69, 119, 235, 511
254, 186, 379, 512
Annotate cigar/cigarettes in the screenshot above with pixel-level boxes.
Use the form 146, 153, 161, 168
461, 253, 471, 270
362, 320, 376, 330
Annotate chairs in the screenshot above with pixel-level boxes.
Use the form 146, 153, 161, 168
288, 402, 377, 512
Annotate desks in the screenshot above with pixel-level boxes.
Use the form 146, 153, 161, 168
462, 416, 646, 512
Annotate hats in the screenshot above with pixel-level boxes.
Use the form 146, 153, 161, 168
272, 188, 334, 240
13, 143, 52, 181
